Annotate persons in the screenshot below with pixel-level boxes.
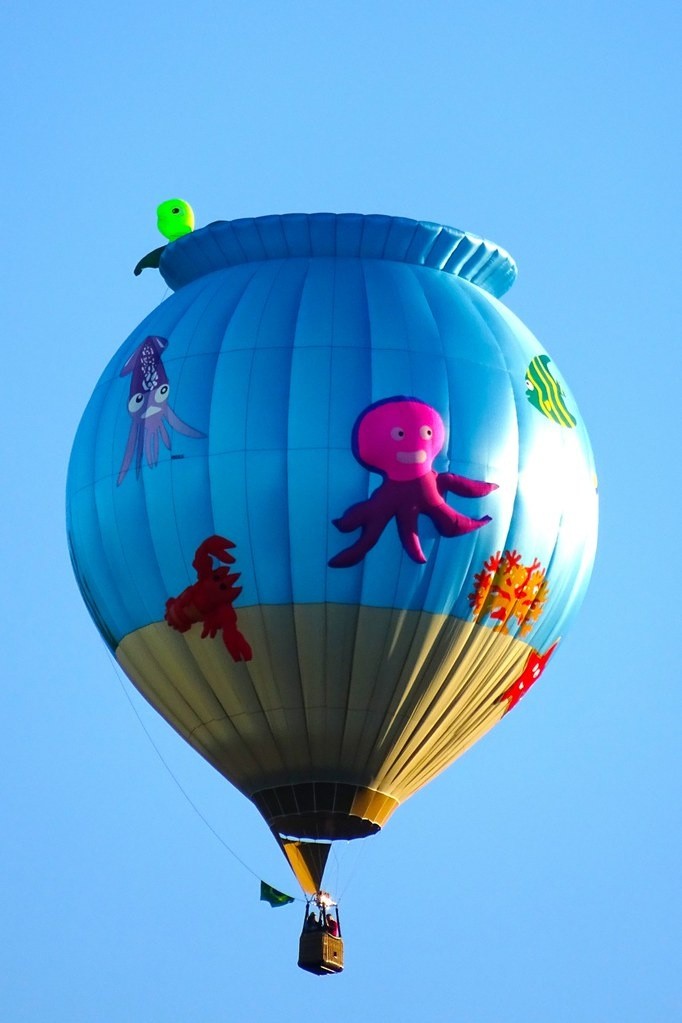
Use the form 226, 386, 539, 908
325, 914, 338, 936
306, 912, 322, 930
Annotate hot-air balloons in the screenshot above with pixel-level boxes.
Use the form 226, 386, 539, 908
62, 198, 601, 977
